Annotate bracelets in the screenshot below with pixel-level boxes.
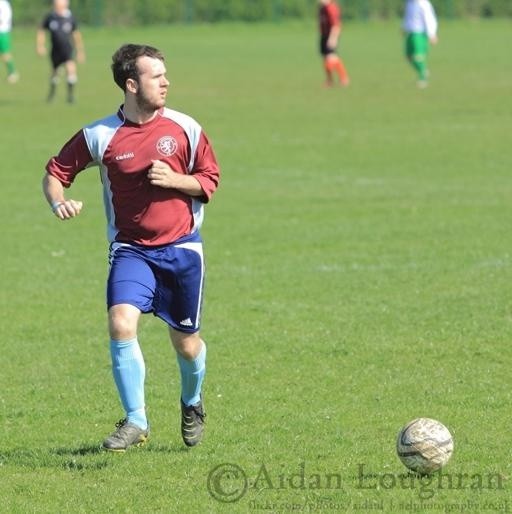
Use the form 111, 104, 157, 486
50, 198, 67, 212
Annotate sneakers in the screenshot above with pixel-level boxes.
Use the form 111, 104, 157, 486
100, 417, 150, 451
180, 391, 205, 448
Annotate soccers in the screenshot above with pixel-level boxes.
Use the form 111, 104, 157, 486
397, 416, 454, 475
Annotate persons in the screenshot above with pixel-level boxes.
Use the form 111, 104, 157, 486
35, 0, 87, 106
399, 0, 440, 91
41, 41, 224, 453
315, 1, 352, 89
0, 0, 20, 85
